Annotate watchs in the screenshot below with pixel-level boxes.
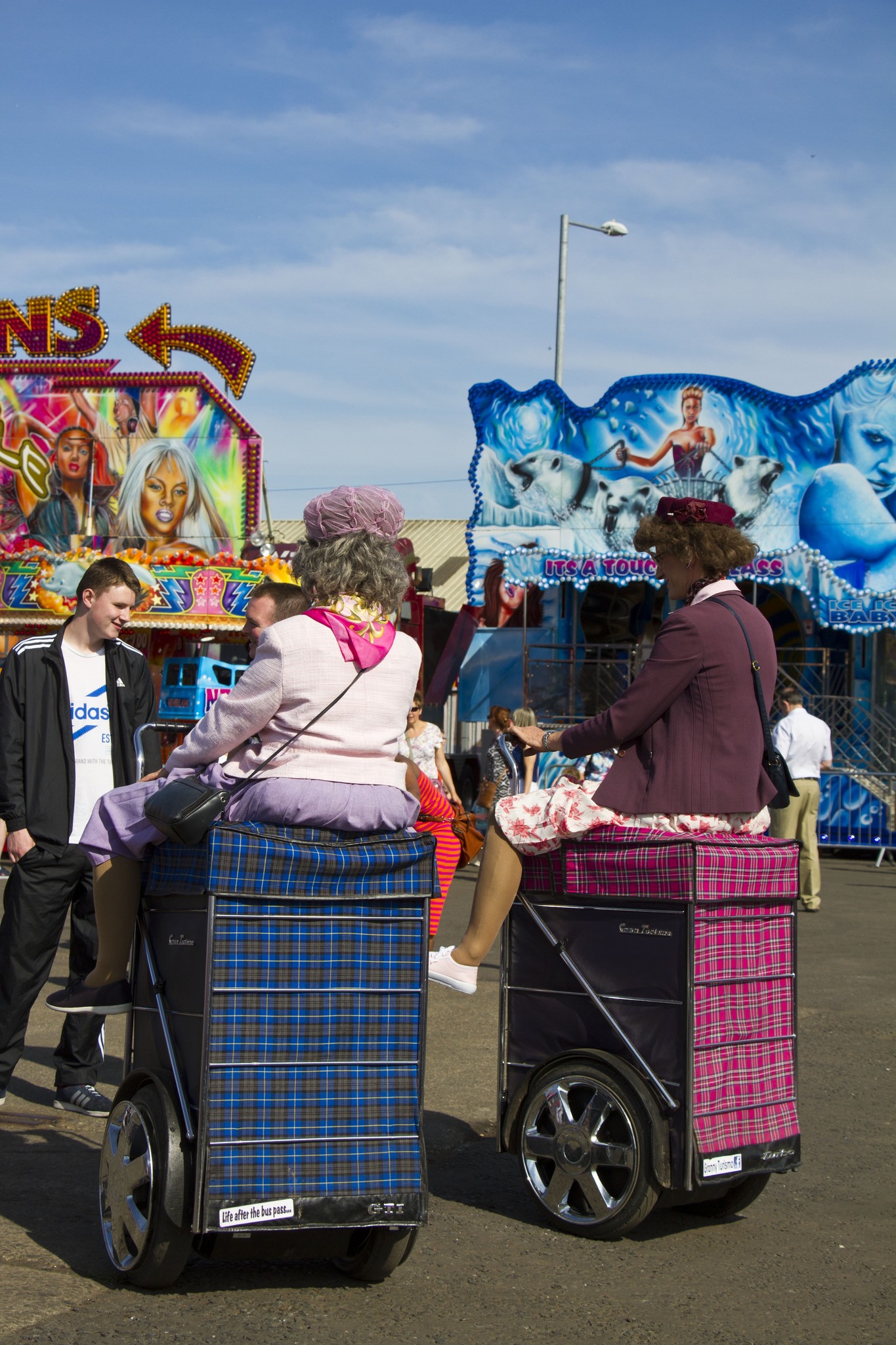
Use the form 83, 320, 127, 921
543, 731, 558, 752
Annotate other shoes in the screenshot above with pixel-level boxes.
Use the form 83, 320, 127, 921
805, 905, 819, 912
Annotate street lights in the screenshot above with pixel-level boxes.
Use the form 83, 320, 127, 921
557, 213, 629, 393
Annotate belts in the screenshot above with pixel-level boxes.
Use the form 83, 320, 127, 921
795, 775, 819, 782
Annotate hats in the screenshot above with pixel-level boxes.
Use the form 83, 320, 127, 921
299, 484, 404, 542
655, 495, 736, 526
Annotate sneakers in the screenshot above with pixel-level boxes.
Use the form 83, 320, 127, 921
51, 1083, 115, 1122
44, 980, 139, 1017
425, 945, 479, 995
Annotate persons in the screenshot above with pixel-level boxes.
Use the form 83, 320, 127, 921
405, 690, 464, 806
427, 493, 796, 995
0, 558, 163, 1118
242, 583, 311, 660
471, 785, 489, 866
44, 483, 424, 1016
508, 707, 540, 794
477, 705, 515, 810
770, 687, 832, 911
576, 746, 617, 784
393, 753, 486, 953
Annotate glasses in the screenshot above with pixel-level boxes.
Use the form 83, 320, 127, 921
652, 547, 671, 569
411, 707, 422, 712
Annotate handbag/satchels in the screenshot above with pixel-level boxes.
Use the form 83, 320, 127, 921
762, 744, 799, 811
477, 778, 496, 810
142, 775, 232, 844
451, 801, 485, 869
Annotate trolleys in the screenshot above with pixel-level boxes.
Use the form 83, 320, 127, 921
497, 731, 803, 1234
83, 709, 430, 1286
